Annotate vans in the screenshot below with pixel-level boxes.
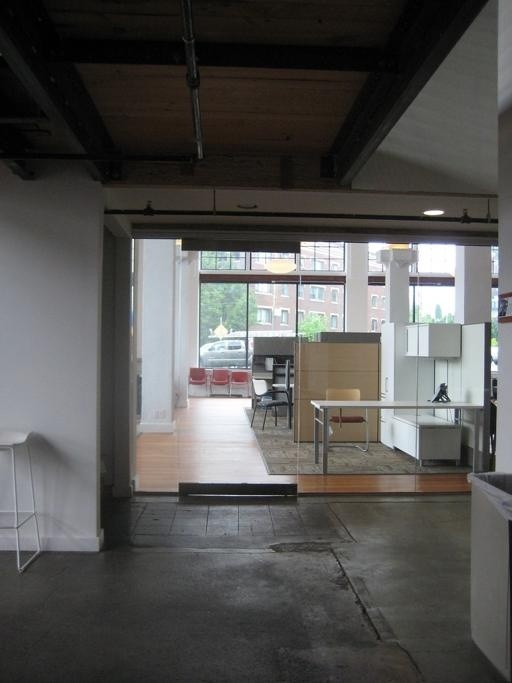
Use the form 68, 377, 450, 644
199, 338, 253, 368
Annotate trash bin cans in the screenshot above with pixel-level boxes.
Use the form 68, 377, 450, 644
467, 471, 512, 682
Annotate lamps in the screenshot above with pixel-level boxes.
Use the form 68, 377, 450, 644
143, 200, 154, 216
460, 209, 472, 224
389, 244, 409, 250
266, 260, 297, 274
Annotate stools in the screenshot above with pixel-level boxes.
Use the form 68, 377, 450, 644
0, 430, 42, 574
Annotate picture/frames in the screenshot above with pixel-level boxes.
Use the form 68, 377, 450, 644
498, 292, 512, 323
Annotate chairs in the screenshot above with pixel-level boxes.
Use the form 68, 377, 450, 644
187, 368, 249, 398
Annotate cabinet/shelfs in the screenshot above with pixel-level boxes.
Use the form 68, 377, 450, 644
251, 322, 491, 468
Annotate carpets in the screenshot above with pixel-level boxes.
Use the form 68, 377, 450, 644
245, 409, 482, 475
